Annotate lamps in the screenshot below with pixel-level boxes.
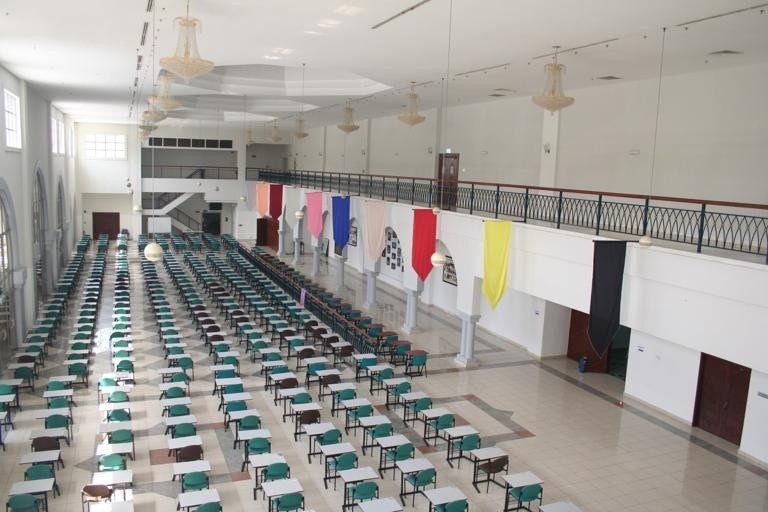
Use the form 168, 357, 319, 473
124, 0, 217, 261
397, 82, 425, 125
529, 46, 573, 113
245, 128, 255, 146
294, 114, 312, 141
630, 38, 665, 249
270, 119, 283, 144
338, 100, 360, 133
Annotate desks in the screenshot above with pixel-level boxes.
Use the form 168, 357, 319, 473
1, 231, 580, 512
